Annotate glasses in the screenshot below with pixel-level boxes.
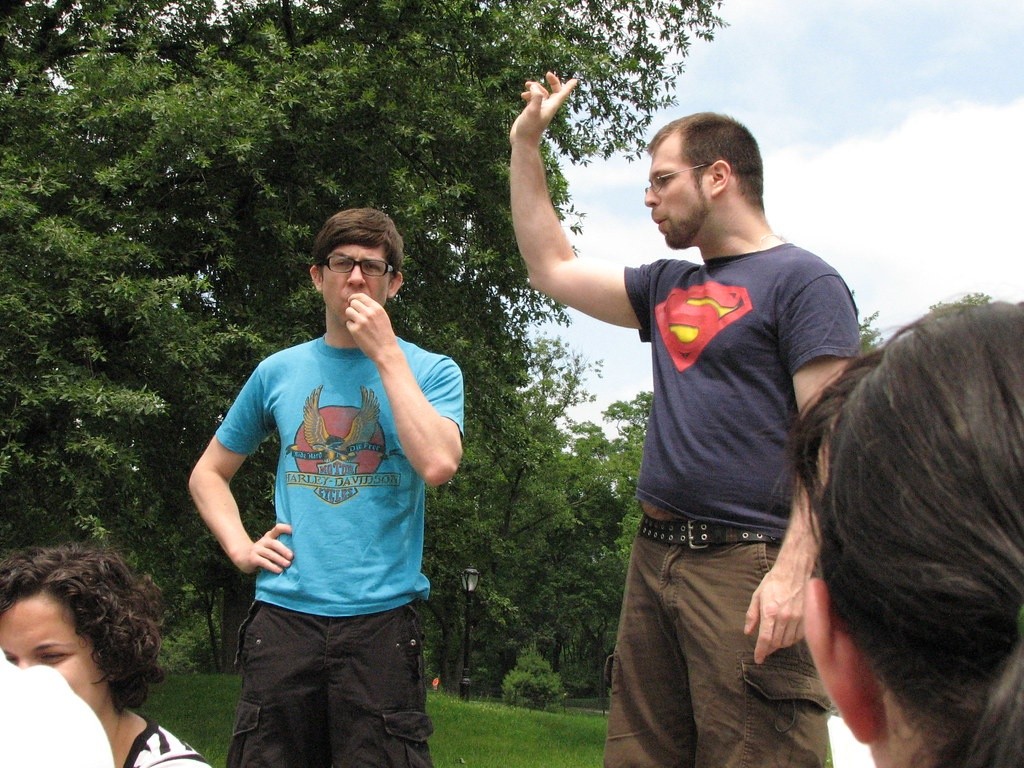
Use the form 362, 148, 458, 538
645, 163, 711, 194
319, 255, 394, 277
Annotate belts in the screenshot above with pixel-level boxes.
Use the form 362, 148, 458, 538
638, 514, 780, 550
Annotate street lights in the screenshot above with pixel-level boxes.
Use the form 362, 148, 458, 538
460, 568, 479, 704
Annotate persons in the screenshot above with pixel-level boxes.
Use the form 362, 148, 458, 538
503, 73, 863, 768
186, 206, 466, 768
0, 543, 213, 768
786, 300, 1024, 768
